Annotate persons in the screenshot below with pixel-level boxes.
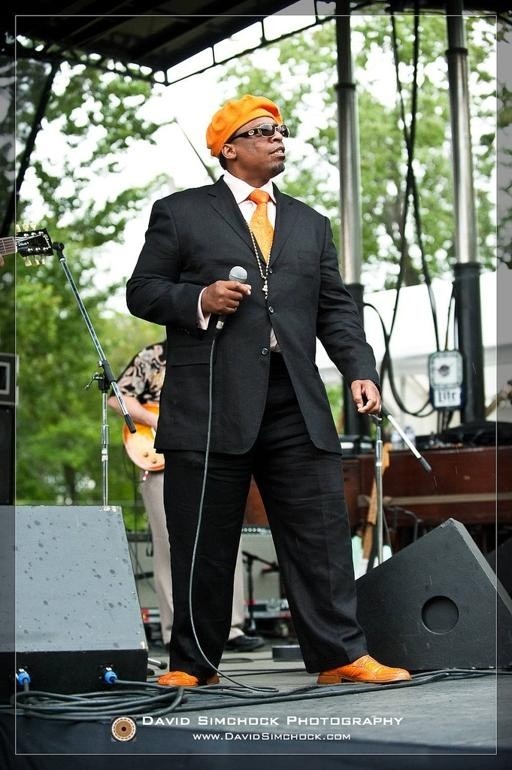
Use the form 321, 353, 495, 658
125, 95, 413, 686
102, 339, 267, 652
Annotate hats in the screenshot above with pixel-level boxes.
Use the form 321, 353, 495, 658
206, 95, 280, 158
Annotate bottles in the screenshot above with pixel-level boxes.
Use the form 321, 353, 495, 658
392, 426, 416, 451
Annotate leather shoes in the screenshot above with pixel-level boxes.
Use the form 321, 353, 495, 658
317, 654, 411, 683
224, 634, 265, 652
158, 669, 220, 686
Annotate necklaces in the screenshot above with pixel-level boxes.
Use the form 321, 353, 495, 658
247, 221, 272, 301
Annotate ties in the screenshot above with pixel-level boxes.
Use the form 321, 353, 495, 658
247, 189, 274, 265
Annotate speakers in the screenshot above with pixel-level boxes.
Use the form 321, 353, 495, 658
356, 516, 512, 673
0, 505, 148, 705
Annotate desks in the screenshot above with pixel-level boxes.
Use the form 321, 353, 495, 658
360, 445, 512, 527
243, 461, 362, 531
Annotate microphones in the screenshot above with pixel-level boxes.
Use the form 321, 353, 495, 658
214, 266, 248, 337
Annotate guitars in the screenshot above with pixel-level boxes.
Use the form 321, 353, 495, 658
351, 443, 392, 580
123, 400, 164, 473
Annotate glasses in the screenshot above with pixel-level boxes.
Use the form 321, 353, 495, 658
226, 124, 289, 144
